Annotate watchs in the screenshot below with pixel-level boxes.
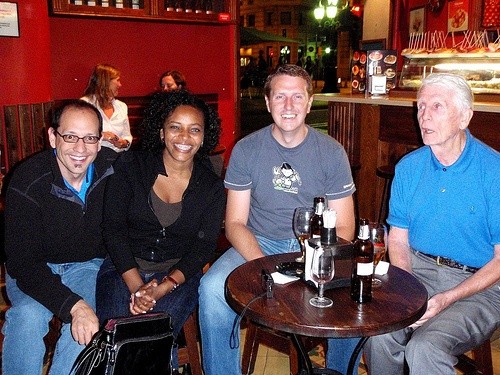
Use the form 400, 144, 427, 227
126, 140, 130, 146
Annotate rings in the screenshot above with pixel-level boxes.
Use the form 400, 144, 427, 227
109, 137, 112, 139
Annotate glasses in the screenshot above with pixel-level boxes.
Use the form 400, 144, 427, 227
55, 129, 100, 144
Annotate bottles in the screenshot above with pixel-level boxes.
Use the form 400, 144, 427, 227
349, 218, 374, 305
311, 197, 325, 238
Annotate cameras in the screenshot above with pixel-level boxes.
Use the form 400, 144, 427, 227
261, 268, 275, 297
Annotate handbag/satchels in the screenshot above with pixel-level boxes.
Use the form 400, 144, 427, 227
68, 312, 173, 375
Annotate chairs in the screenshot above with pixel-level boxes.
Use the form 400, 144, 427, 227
375, 165, 493, 375
240, 162, 361, 375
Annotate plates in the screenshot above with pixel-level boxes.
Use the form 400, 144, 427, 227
352, 51, 397, 91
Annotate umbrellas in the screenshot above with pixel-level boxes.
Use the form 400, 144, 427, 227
239, 27, 304, 45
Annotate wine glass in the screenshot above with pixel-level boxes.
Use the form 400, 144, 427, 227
368, 224, 388, 288
294, 207, 316, 263
309, 245, 334, 307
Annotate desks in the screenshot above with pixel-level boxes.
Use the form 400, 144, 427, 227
223, 252, 428, 375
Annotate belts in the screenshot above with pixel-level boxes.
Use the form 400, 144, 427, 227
419, 251, 478, 274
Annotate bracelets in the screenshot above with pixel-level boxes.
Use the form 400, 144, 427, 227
162, 276, 179, 293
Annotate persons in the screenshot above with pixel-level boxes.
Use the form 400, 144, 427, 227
198, 65, 361, 375
246, 50, 268, 75
0, 99, 119, 375
297, 53, 339, 93
81, 64, 133, 153
95, 90, 225, 375
160, 71, 186, 91
277, 56, 284, 66
364, 73, 500, 375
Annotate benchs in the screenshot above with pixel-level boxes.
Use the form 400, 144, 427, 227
2, 93, 226, 175
